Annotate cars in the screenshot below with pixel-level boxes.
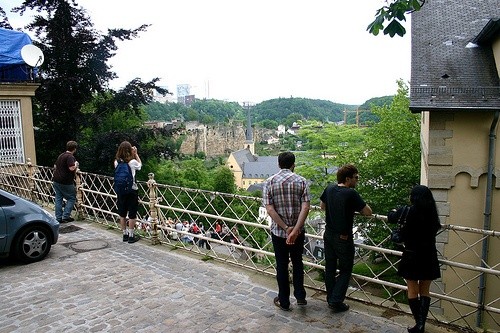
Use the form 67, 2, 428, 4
0, 188, 60, 263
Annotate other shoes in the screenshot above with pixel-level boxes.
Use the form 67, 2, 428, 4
123, 232, 130, 241
274, 297, 289, 311
58, 220, 64, 223
329, 303, 335, 309
62, 217, 74, 222
297, 298, 307, 305
129, 235, 140, 242
335, 303, 349, 313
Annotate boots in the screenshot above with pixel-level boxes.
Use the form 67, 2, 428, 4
407, 295, 431, 333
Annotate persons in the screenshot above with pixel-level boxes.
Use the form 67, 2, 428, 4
114, 142, 143, 243
53, 141, 79, 223
265, 152, 311, 309
157, 217, 239, 252
321, 164, 372, 311
125, 215, 151, 239
387, 185, 442, 333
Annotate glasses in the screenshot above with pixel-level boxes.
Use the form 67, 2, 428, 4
350, 175, 359, 181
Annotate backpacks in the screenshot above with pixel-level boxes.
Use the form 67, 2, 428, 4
113, 159, 133, 194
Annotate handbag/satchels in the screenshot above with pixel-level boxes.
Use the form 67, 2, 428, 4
390, 206, 410, 243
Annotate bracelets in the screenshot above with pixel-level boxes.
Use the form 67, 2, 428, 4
285, 226, 288, 231
